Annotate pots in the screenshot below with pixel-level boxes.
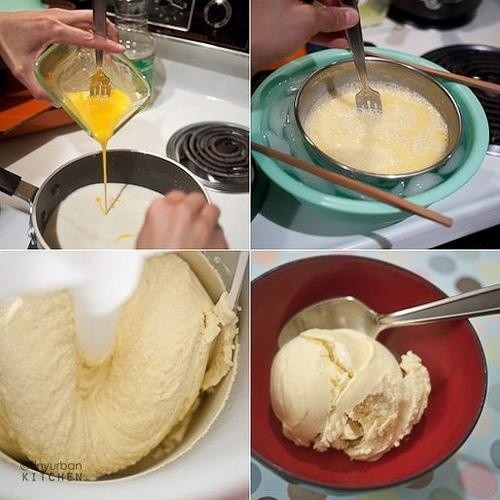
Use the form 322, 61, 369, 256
1, 148, 213, 250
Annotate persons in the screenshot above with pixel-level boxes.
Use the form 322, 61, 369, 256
252, 0, 360, 77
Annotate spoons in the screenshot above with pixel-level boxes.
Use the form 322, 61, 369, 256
275, 280, 500, 355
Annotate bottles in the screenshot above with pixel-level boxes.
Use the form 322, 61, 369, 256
116, 0, 156, 112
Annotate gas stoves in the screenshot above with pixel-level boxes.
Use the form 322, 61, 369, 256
250, 1, 499, 250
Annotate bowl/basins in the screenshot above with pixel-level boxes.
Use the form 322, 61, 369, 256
291, 56, 464, 191
251, 252, 489, 493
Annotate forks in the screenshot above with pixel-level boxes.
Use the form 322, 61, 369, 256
335, 0, 383, 115
88, 1, 114, 100
